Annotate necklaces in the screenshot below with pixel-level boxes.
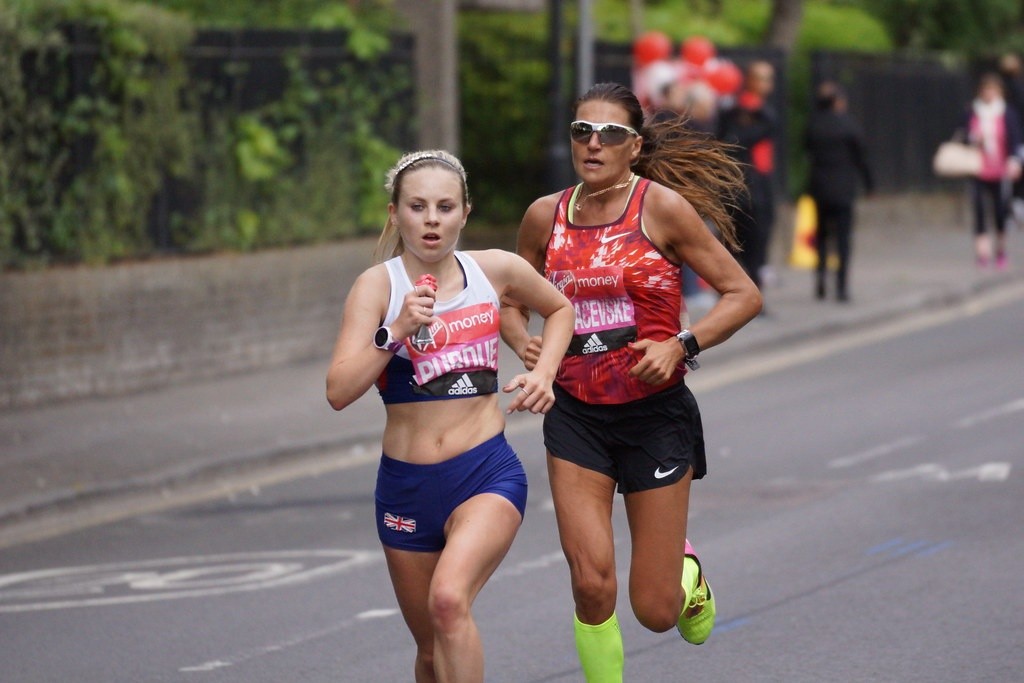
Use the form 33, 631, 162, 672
574, 172, 634, 212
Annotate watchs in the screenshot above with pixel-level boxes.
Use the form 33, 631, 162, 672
373, 326, 402, 354
677, 330, 701, 371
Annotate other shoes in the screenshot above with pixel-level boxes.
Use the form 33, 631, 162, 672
977, 252, 991, 270
995, 251, 1010, 274
837, 285, 847, 305
817, 278, 826, 299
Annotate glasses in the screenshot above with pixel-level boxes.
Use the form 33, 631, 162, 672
571, 120, 638, 145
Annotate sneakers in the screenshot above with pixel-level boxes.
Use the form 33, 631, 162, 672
676, 537, 716, 646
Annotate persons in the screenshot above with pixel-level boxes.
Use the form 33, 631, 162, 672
499, 83, 763, 683
959, 52, 1024, 271
650, 60, 873, 304
326, 149, 575, 683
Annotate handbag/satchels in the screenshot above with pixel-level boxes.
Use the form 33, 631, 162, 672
932, 140, 985, 179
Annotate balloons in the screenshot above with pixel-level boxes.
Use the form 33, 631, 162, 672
633, 30, 743, 122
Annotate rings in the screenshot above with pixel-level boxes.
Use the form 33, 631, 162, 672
414, 286, 419, 297
522, 389, 529, 396
424, 306, 425, 315
